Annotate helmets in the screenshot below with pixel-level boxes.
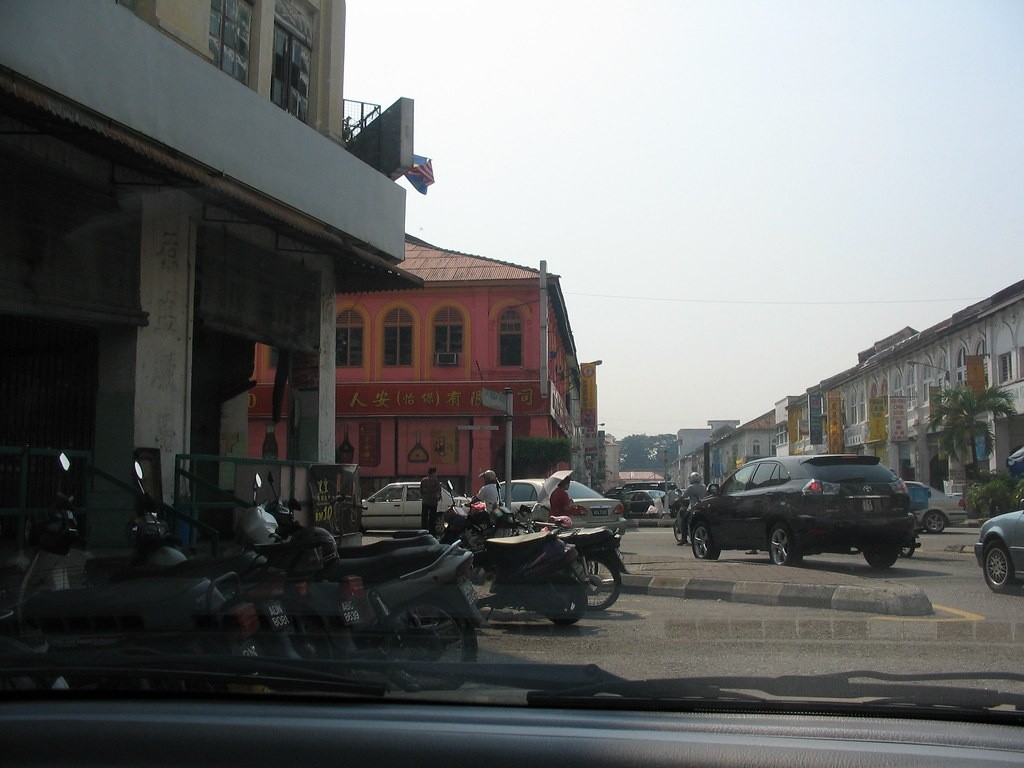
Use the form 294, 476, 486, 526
689, 472, 701, 484
483, 469, 496, 482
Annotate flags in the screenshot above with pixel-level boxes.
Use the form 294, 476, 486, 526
406, 153, 434, 193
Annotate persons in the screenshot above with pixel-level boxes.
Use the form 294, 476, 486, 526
548, 476, 574, 516
676, 472, 707, 546
667, 482, 678, 517
457, 470, 501, 547
420, 467, 442, 536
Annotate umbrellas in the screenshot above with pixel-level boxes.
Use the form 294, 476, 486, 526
537, 470, 574, 504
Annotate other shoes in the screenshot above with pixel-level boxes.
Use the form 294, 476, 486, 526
677, 538, 688, 546
745, 551, 758, 555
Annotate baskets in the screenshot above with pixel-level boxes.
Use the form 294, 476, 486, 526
668, 505, 679, 514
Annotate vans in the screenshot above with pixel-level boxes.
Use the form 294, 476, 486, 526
620, 481, 679, 493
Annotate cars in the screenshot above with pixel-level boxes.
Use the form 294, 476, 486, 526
901, 480, 968, 534
603, 487, 623, 499
973, 509, 1024, 592
362, 482, 453, 533
620, 490, 666, 513
460, 481, 626, 537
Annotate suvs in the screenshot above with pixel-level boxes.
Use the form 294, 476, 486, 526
688, 456, 916, 567
105, 458, 398, 700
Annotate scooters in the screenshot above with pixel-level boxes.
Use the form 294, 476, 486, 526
210, 471, 421, 695
262, 472, 488, 693
436, 479, 598, 625
0, 451, 272, 713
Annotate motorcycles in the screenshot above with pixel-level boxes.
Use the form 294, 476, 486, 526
461, 493, 631, 611
672, 497, 696, 545
